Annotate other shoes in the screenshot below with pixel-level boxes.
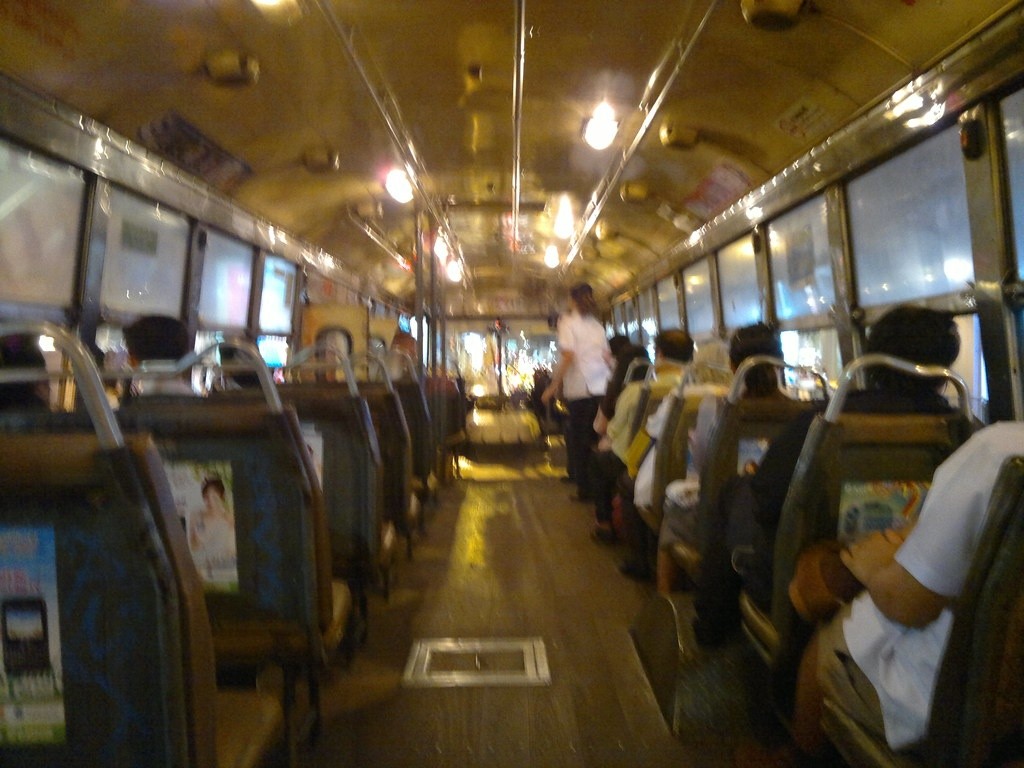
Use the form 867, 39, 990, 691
588, 523, 616, 544
730, 739, 799, 768
616, 554, 655, 584
786, 542, 857, 624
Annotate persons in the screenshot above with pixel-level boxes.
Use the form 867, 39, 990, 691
220, 340, 261, 389
1, 335, 49, 411
189, 477, 236, 572
313, 320, 463, 385
539, 282, 618, 488
566, 314, 786, 598
122, 315, 197, 397
734, 420, 1024, 768
685, 303, 962, 653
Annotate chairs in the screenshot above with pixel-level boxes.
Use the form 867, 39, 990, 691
389, 359, 468, 487
77, 336, 350, 768
301, 350, 413, 572
658, 355, 831, 649
0, 318, 278, 768
814, 456, 1024, 767
353, 353, 432, 539
736, 356, 977, 708
201, 339, 385, 608
616, 356, 736, 592
